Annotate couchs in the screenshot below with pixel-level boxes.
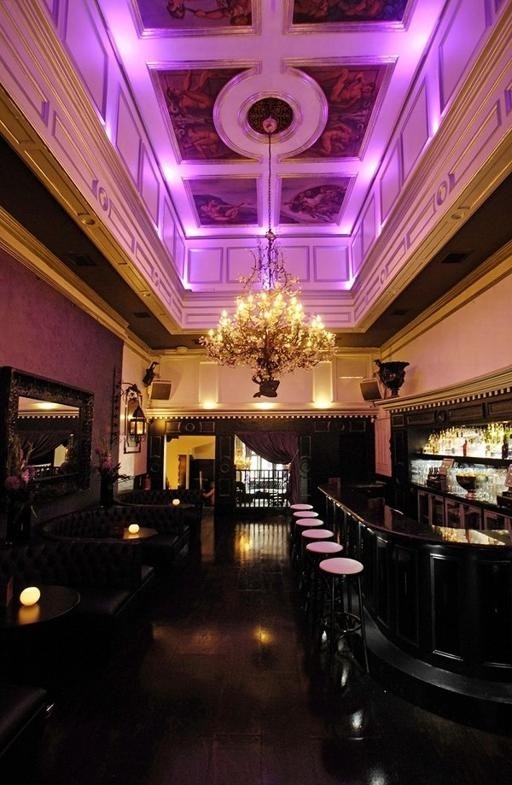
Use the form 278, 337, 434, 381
0, 490, 203, 784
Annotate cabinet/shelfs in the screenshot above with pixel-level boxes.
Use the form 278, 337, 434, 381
408, 419, 512, 530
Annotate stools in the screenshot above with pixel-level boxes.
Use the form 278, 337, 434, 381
289, 503, 372, 700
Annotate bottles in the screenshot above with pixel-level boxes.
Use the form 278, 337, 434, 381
422, 421, 512, 460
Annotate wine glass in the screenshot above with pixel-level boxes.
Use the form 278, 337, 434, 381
409, 458, 508, 505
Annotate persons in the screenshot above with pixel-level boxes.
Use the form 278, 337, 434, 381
203, 481, 215, 505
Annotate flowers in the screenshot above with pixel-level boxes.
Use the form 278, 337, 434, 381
1, 432, 41, 494
92, 436, 134, 484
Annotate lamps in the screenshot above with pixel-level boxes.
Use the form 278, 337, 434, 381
121, 382, 148, 445
195, 115, 338, 381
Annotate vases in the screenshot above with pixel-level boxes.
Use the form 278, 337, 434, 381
100, 482, 113, 510
4, 490, 32, 547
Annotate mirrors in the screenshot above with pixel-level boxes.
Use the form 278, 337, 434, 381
1, 365, 95, 501
